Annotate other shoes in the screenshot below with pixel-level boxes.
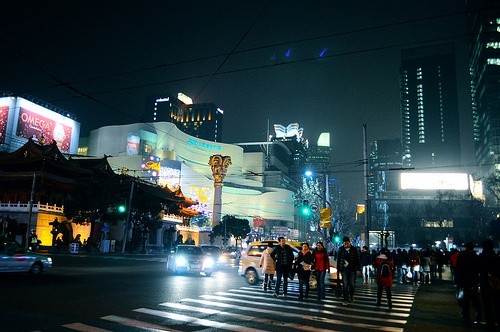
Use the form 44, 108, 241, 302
350, 295, 353, 302
473, 318, 487, 324
343, 300, 349, 305
274, 294, 278, 297
283, 292, 287, 298
264, 284, 267, 291
268, 284, 272, 290
299, 295, 303, 299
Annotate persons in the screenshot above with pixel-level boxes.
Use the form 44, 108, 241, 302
392, 240, 449, 286
360, 246, 372, 284
294, 243, 313, 298
28, 229, 37, 251
449, 239, 500, 332
271, 237, 294, 297
330, 248, 337, 260
56, 237, 62, 244
311, 242, 329, 299
259, 242, 276, 291
337, 237, 360, 305
7, 231, 16, 240
371, 249, 378, 273
375, 248, 393, 308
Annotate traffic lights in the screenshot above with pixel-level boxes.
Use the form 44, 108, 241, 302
301, 199, 310, 219
119, 198, 127, 214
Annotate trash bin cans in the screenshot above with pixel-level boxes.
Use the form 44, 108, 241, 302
110, 240, 116, 252
100, 239, 110, 253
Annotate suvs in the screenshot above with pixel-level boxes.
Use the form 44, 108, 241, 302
238, 240, 343, 291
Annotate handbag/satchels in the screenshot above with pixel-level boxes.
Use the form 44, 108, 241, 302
335, 272, 341, 298
304, 263, 309, 271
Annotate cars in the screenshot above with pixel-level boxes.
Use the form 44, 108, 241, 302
0, 236, 53, 277
166, 244, 241, 276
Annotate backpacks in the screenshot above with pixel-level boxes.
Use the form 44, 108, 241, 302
381, 260, 390, 277
